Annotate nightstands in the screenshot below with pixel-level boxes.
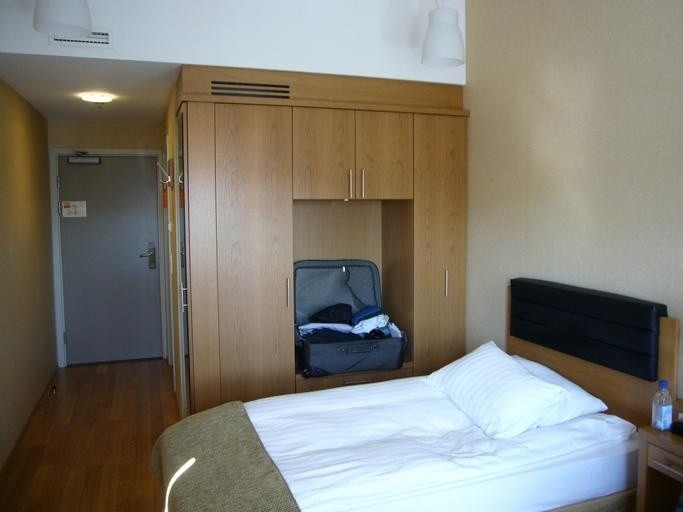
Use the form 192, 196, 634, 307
632, 417, 683, 512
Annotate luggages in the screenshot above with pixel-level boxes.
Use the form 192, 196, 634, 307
294, 259, 407, 379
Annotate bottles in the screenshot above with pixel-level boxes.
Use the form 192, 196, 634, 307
649, 379, 673, 431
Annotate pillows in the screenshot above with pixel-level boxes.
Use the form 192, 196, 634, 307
509, 353, 608, 430
429, 339, 570, 440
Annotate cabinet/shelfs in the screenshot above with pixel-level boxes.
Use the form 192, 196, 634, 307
187, 97, 470, 415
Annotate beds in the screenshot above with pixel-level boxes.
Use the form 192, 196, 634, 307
150, 277, 680, 511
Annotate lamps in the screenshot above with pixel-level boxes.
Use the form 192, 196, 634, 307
32, 1, 93, 39
